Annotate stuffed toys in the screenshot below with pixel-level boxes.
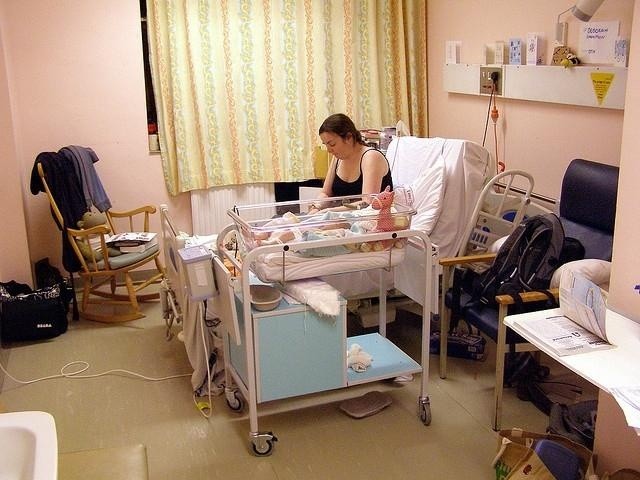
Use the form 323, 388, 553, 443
361, 185, 409, 252
77, 212, 121, 262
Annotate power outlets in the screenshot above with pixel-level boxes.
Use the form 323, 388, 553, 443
480, 67, 503, 95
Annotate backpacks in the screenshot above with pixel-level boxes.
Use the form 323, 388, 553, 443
473, 213, 567, 309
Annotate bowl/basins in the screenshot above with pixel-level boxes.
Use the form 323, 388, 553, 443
250, 284, 283, 312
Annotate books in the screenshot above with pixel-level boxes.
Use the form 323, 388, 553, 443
513, 264, 618, 357
106, 232, 158, 253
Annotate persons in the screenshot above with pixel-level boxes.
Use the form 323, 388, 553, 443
241, 113, 393, 246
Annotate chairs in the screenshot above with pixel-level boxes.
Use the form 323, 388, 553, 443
439, 159, 619, 431
37, 150, 168, 324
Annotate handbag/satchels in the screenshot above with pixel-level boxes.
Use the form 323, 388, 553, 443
430, 332, 486, 360
490, 427, 640, 480
0, 280, 67, 340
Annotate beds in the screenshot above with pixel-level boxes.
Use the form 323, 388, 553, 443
159, 138, 490, 398
